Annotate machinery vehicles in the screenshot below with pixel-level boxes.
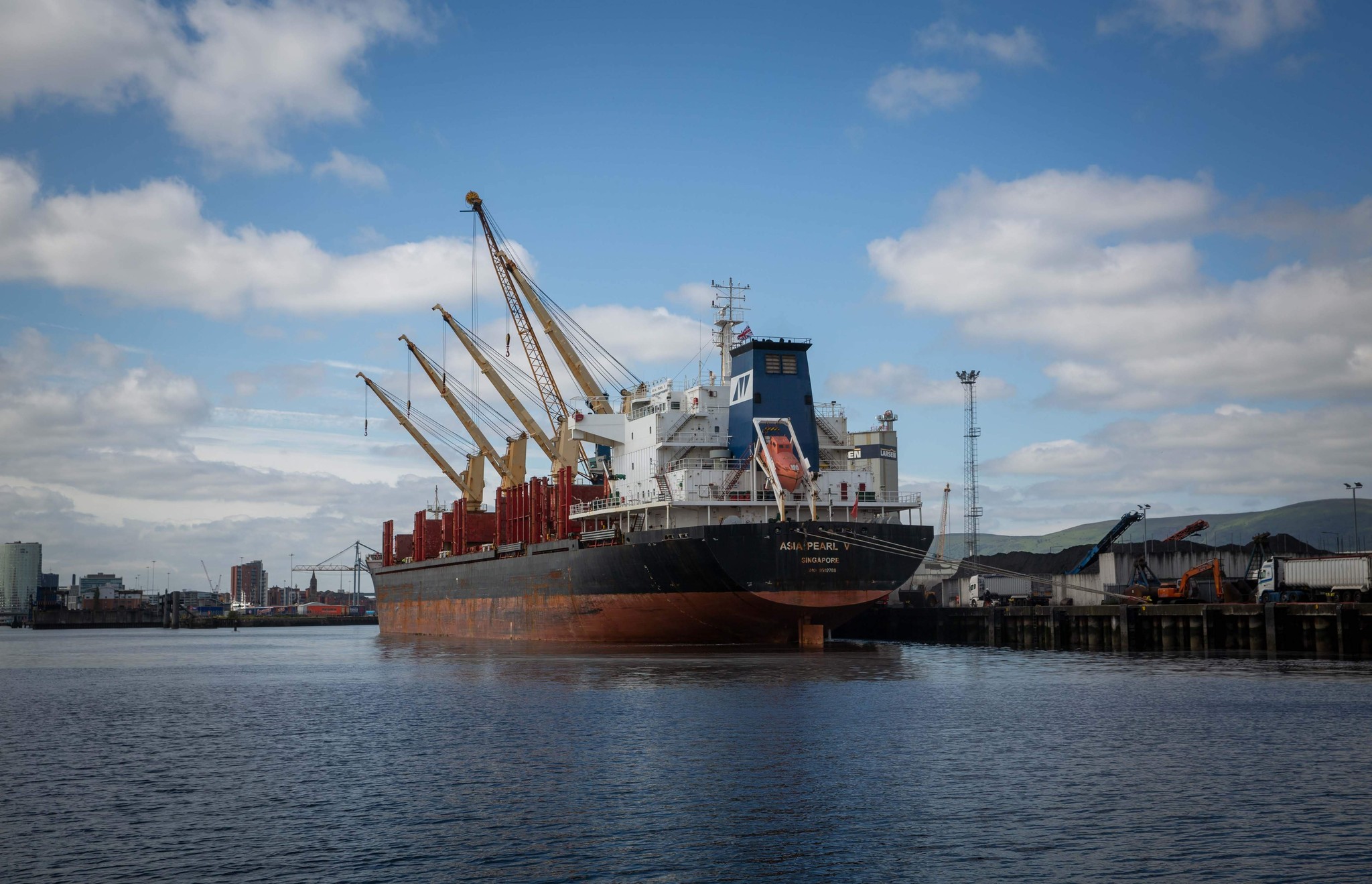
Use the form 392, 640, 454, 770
1157, 558, 1225, 604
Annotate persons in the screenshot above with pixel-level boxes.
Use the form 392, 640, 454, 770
984, 588, 992, 606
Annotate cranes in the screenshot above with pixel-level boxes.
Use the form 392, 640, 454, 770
355, 366, 487, 507
393, 333, 530, 491
430, 302, 581, 483
201, 560, 222, 594
936, 481, 952, 560
292, 540, 384, 572
458, 188, 594, 485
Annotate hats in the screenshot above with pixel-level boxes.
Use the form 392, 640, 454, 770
986, 588, 989, 590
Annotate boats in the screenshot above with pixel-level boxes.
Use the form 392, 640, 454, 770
363, 273, 932, 651
764, 438, 804, 495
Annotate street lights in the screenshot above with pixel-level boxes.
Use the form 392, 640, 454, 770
1137, 503, 1151, 575
166, 572, 171, 593
152, 560, 157, 595
239, 557, 245, 602
1321, 531, 1339, 553
134, 574, 141, 590
284, 580, 286, 587
146, 566, 150, 602
1343, 481, 1363, 555
288, 553, 295, 605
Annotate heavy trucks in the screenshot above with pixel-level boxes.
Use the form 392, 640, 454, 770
1256, 555, 1372, 604
968, 573, 1053, 607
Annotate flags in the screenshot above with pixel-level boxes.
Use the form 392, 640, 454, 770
851, 494, 859, 519
737, 324, 755, 341
